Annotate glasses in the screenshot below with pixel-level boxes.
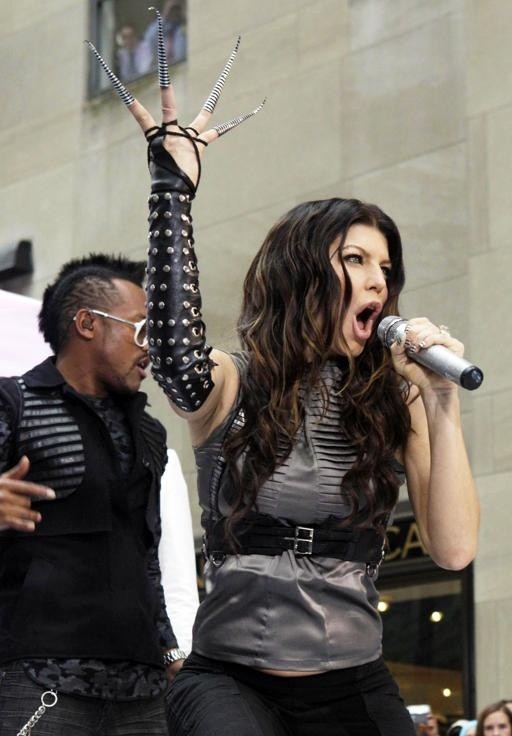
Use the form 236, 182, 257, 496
73, 309, 148, 349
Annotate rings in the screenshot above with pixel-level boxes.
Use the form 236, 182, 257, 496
438, 324, 449, 335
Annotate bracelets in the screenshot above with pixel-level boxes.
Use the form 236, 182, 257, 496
162, 649, 187, 669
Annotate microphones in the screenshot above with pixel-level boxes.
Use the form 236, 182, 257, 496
377, 315, 484, 391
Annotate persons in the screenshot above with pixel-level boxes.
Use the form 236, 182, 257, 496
111, 1, 175, 81
161, 4, 185, 66
1, 251, 186, 734
79, 6, 482, 734
404, 695, 512, 735
